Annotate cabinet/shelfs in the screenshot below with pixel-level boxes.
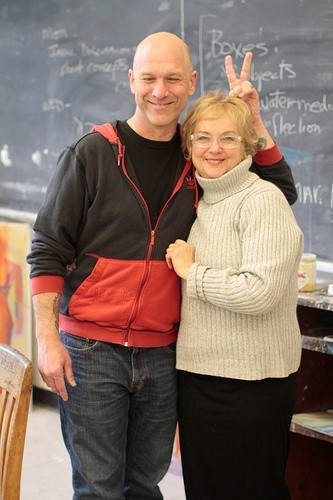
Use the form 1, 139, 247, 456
290, 285, 333, 500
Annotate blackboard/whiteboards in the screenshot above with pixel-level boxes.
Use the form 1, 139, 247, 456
0, 0, 333, 273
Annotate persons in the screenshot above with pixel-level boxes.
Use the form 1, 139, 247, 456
66, 90, 304, 500
26, 31, 298, 500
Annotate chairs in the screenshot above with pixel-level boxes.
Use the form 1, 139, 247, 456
0, 344, 35, 500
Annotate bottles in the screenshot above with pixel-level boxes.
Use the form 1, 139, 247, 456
298, 253, 316, 292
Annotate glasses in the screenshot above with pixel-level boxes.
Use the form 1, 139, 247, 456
189, 132, 244, 149
